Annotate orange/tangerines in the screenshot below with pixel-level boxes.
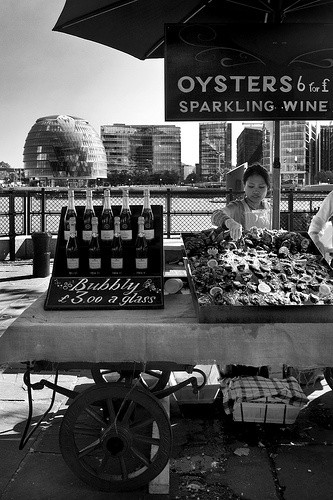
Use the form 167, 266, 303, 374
198, 238, 331, 303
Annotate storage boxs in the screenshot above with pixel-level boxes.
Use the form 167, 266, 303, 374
231, 398, 302, 447
179, 229, 333, 325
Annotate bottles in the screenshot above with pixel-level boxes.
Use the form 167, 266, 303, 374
100, 189, 115, 252
82, 190, 96, 249
119, 189, 134, 246
65, 217, 80, 277
141, 189, 154, 244
134, 216, 150, 275
88, 217, 103, 275
63, 189, 79, 250
110, 216, 124, 275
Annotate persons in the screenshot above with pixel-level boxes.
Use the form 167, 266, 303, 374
308, 190, 333, 258
212, 165, 273, 241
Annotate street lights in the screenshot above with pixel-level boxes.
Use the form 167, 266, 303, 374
159, 179, 162, 187
98, 179, 100, 186
129, 179, 131, 186
219, 153, 224, 175
191, 179, 193, 186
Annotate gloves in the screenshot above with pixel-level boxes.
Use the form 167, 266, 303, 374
226, 219, 243, 241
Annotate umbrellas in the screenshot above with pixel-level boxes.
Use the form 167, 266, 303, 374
51, 0, 333, 230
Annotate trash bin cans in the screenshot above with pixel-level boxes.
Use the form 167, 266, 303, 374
32, 232, 51, 277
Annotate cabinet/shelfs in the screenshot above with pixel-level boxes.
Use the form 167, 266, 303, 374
42, 203, 165, 310
